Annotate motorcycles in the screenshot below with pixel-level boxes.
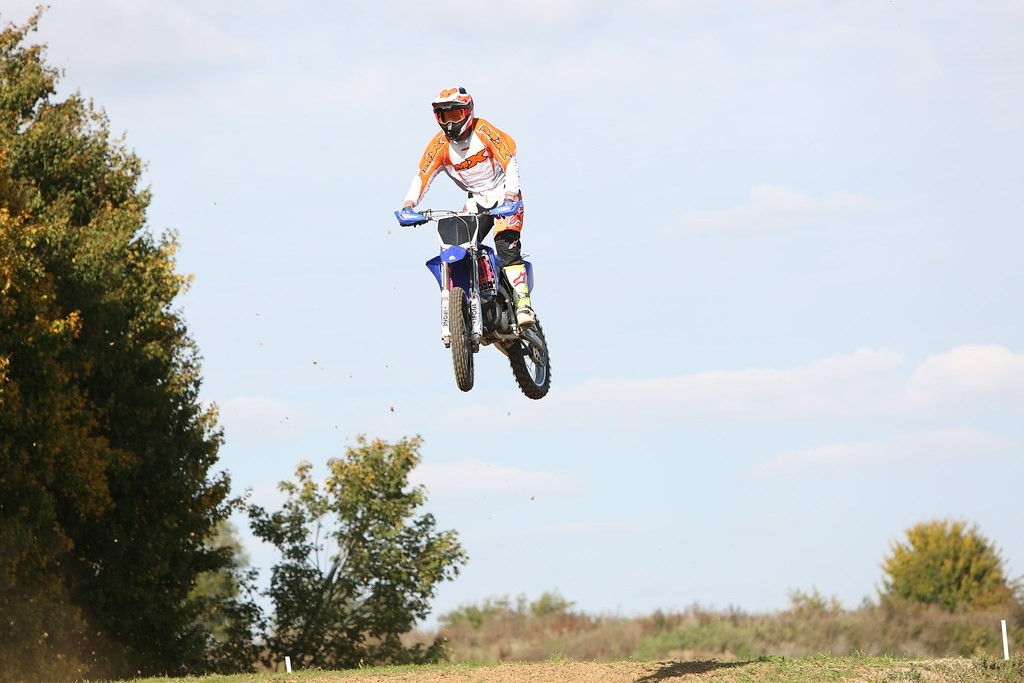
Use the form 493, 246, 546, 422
394, 199, 552, 400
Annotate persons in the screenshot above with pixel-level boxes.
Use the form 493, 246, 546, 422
400, 88, 538, 329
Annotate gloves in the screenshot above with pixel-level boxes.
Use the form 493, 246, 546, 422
403, 206, 415, 214
493, 198, 516, 219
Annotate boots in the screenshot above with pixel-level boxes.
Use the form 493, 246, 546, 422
502, 264, 536, 331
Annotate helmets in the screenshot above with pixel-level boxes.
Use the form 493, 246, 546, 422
432, 86, 474, 141
